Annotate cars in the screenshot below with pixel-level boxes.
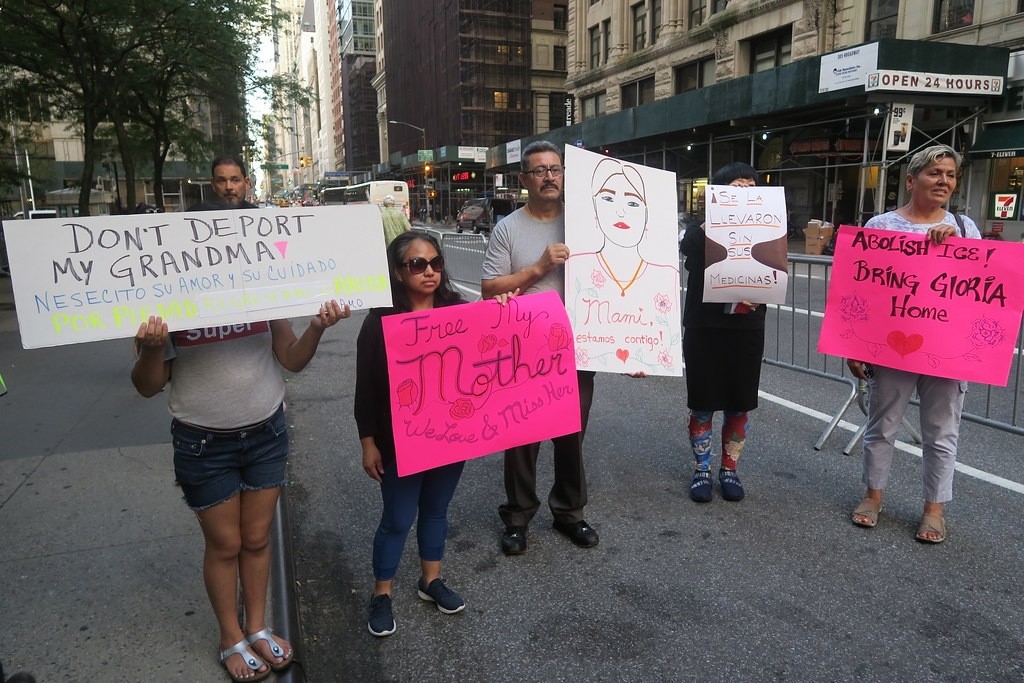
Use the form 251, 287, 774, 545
267, 196, 319, 207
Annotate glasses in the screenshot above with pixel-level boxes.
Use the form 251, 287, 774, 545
402, 256, 445, 274
523, 165, 563, 179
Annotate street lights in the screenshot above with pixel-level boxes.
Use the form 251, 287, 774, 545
388, 121, 433, 228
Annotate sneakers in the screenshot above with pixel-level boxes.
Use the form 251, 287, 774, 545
366, 592, 397, 636
718, 468, 745, 502
553, 518, 600, 548
690, 468, 713, 504
501, 526, 530, 555
417, 577, 465, 614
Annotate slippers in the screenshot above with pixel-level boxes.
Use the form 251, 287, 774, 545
915, 512, 946, 544
851, 498, 883, 527
218, 639, 271, 683
246, 626, 293, 671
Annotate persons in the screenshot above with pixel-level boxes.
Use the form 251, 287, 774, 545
479, 141, 599, 556
353, 233, 521, 637
380, 194, 409, 245
681, 163, 769, 504
187, 155, 262, 211
847, 145, 981, 542
131, 205, 352, 683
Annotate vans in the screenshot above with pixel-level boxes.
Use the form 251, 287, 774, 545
454, 197, 515, 236
13, 208, 80, 219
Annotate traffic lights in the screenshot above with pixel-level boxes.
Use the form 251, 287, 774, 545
299, 157, 305, 168
424, 161, 431, 173
428, 190, 437, 197
307, 158, 312, 168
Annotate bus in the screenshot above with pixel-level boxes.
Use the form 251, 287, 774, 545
343, 180, 412, 224
320, 185, 346, 207
